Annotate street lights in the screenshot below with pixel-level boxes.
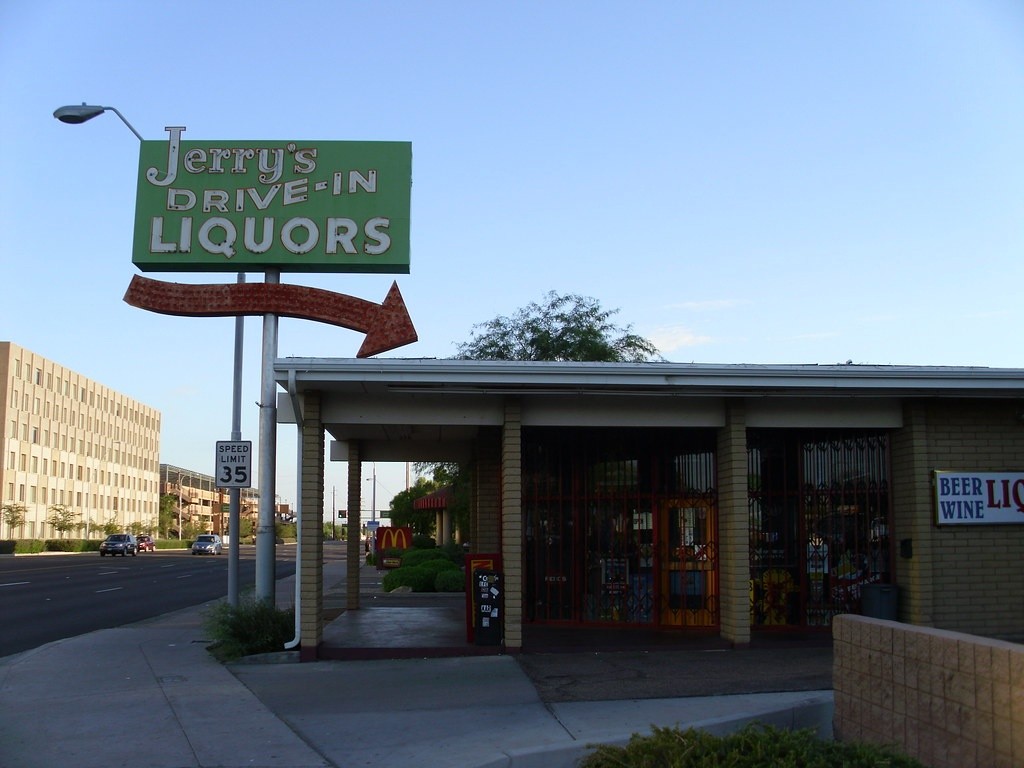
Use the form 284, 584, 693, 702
178, 473, 199, 542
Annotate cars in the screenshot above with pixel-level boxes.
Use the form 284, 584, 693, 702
136, 534, 156, 552
192, 534, 223, 556
98, 533, 138, 557
